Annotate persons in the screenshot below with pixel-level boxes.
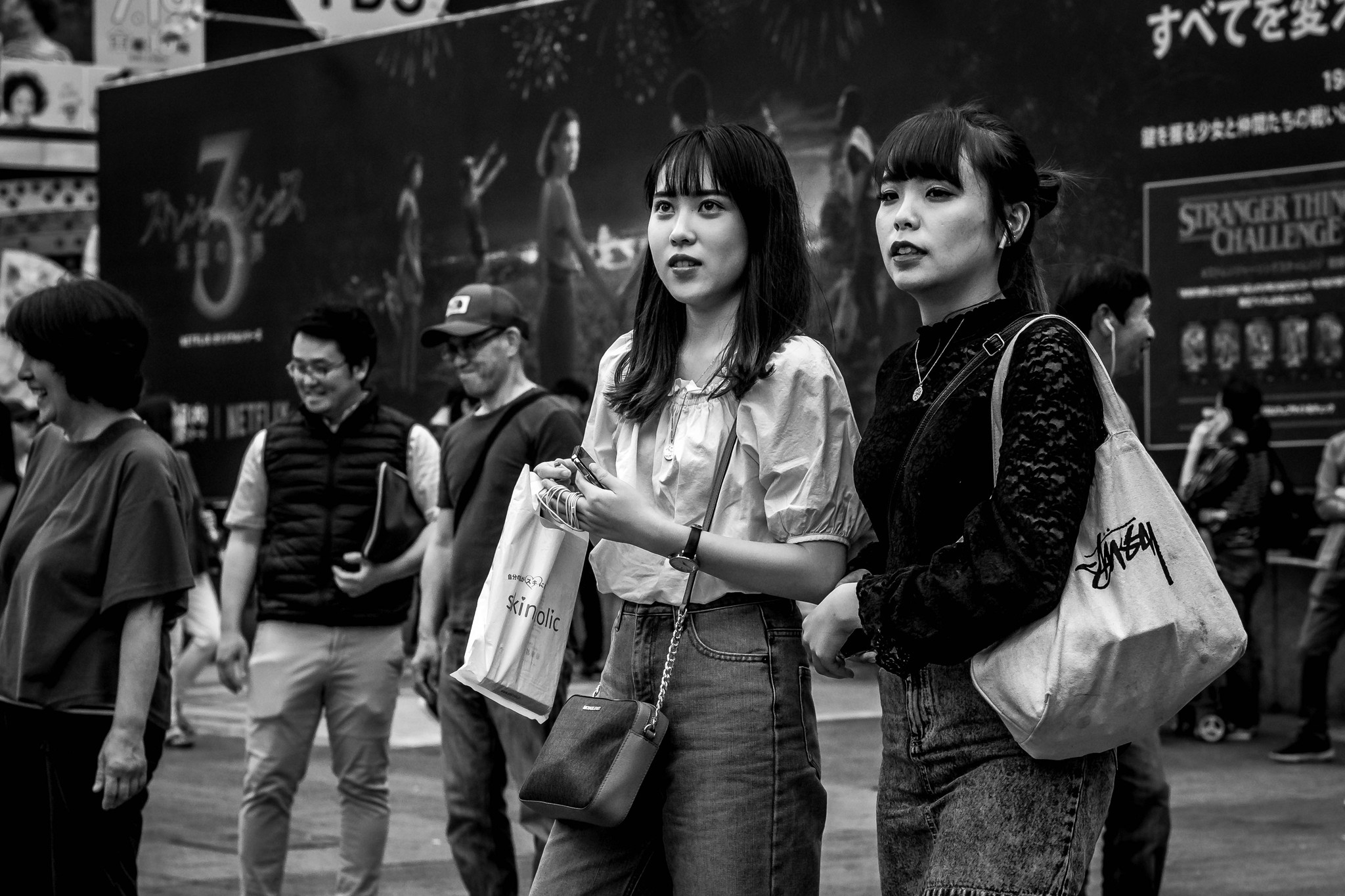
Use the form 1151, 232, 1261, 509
429, 385, 481, 426
411, 284, 581, 896
527, 123, 865, 896
799, 105, 1121, 896
0, 277, 184, 896
211, 300, 443, 896
1178, 372, 1283, 742
576, 541, 606, 676
549, 377, 590, 415
1054, 253, 1172, 896
1270, 432, 1345, 759
0, 398, 43, 539
136, 392, 223, 747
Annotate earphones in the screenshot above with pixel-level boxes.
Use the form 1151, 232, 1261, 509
1102, 318, 1115, 332
998, 219, 1012, 249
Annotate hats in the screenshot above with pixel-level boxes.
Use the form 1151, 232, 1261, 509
419, 282, 531, 349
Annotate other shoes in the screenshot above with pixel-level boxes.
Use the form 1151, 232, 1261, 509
1268, 728, 1335, 762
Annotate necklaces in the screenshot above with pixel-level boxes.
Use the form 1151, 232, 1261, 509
662, 341, 731, 462
912, 317, 967, 402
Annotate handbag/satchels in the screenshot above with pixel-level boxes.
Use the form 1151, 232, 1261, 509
518, 693, 669, 826
970, 313, 1248, 761
449, 463, 590, 726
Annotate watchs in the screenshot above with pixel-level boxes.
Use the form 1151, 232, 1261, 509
668, 524, 703, 573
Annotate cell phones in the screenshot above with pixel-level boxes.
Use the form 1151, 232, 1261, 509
1205, 410, 1233, 445
839, 627, 874, 660
571, 445, 608, 491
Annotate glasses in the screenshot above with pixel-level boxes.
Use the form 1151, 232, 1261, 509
287, 358, 348, 376
441, 326, 508, 363
